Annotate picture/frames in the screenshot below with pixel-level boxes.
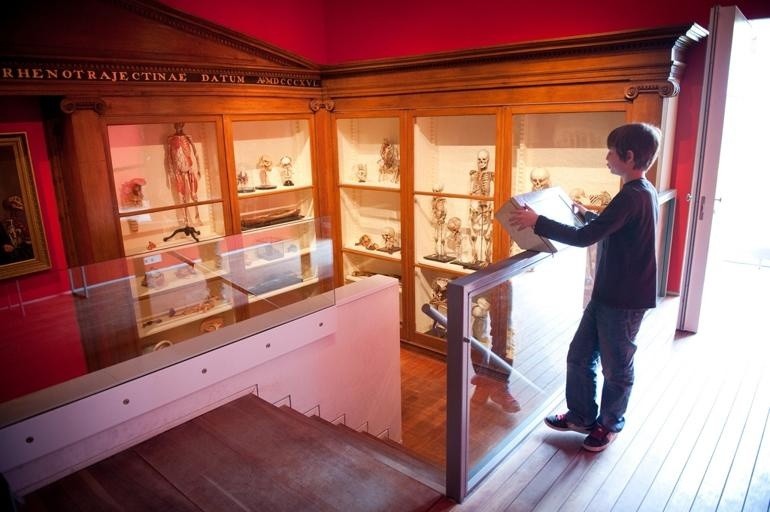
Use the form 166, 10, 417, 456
0, 131, 53, 278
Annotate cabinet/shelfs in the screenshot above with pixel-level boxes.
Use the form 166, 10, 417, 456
58, 20, 335, 369
506, 31, 681, 259
336, 45, 506, 370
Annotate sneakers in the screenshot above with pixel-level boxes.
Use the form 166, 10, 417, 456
471, 384, 521, 413
543, 413, 595, 434
581, 416, 618, 452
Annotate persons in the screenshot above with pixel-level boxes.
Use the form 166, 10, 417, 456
507, 121, 665, 453
